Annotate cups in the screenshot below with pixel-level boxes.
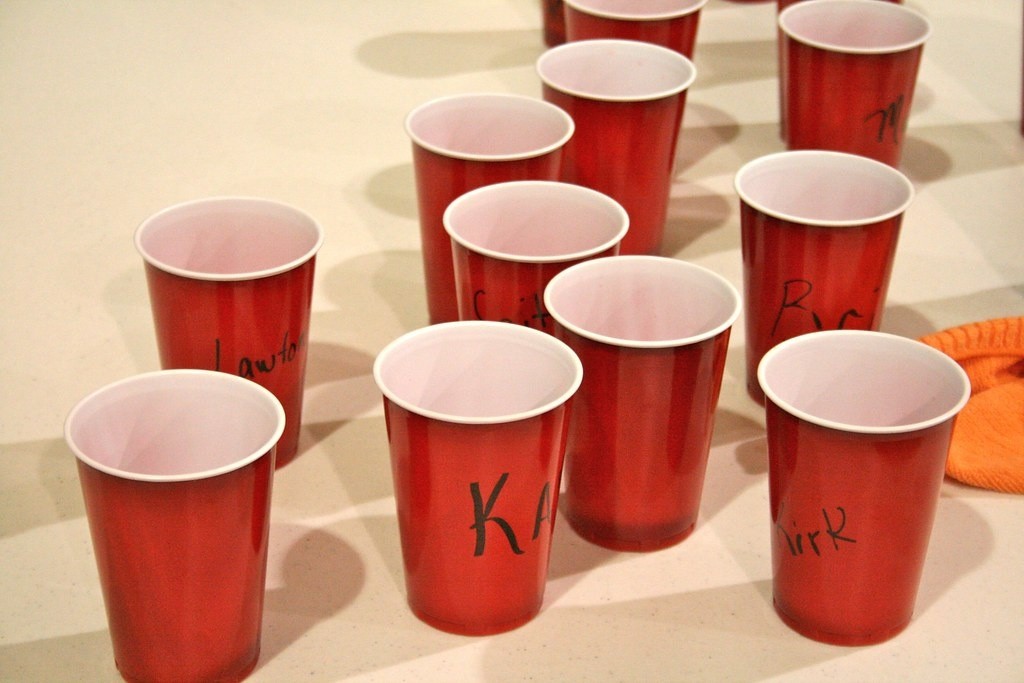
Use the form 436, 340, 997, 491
406, 92, 576, 326
374, 320, 583, 637
778, 0, 933, 170
538, 38, 698, 257
443, 180, 629, 337
540, 0, 566, 49
543, 254, 742, 552
63, 368, 286, 683
564, 0, 707, 62
756, 329, 972, 647
735, 151, 914, 408
136, 196, 326, 470
